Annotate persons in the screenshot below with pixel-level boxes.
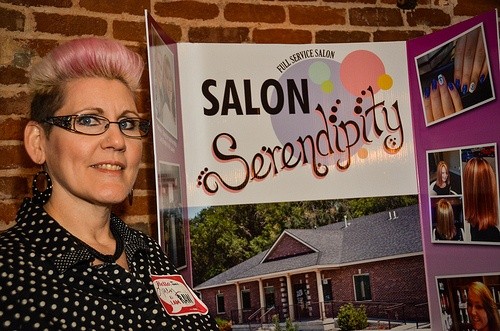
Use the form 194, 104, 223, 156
432, 199, 463, 241
430, 161, 452, 196
423, 27, 488, 124
463, 157, 500, 242
455, 282, 500, 331
0, 37, 219, 330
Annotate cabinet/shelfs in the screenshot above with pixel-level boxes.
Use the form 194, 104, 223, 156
438, 276, 500, 331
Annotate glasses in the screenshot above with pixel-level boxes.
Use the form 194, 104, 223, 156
37, 113, 151, 139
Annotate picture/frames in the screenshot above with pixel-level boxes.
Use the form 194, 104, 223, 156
426, 142, 500, 246
435, 272, 500, 331
414, 22, 496, 127
